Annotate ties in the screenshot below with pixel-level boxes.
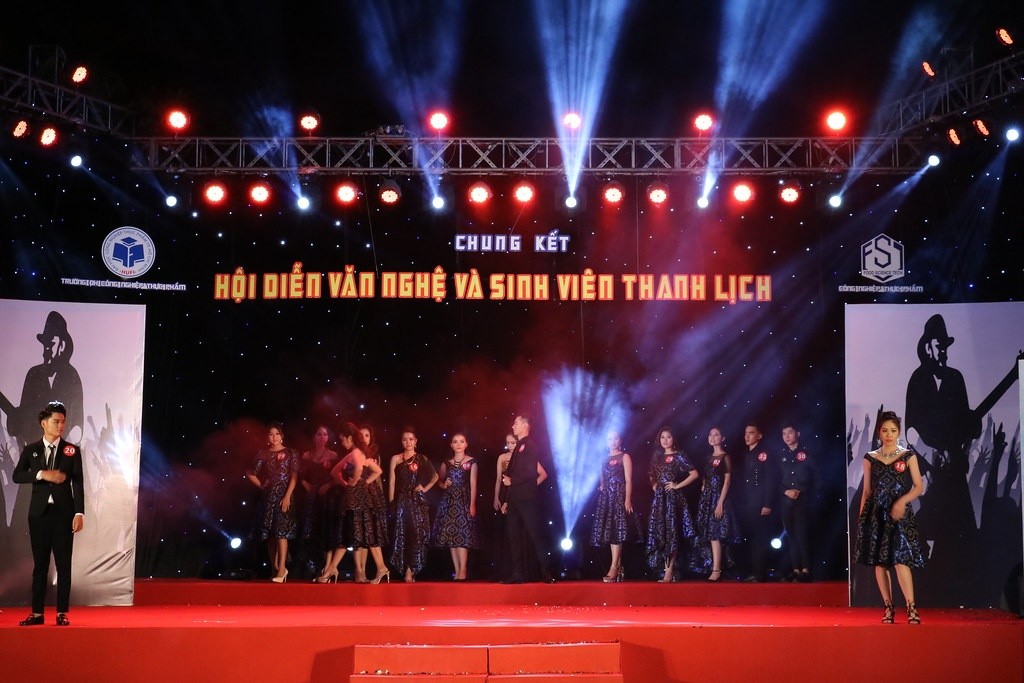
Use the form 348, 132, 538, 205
48, 445, 56, 469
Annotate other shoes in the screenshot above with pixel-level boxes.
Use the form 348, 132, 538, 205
454, 578, 457, 581
458, 576, 468, 582
792, 571, 803, 582
800, 572, 812, 583
357, 575, 369, 583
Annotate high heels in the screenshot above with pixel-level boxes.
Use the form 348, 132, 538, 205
883, 599, 895, 624
272, 569, 288, 583
603, 566, 619, 582
658, 570, 676, 582
404, 573, 416, 583
705, 571, 721, 582
318, 570, 339, 584
618, 566, 625, 582
370, 569, 390, 585
907, 599, 920, 624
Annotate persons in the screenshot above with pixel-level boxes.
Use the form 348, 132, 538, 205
301, 424, 338, 582
248, 424, 297, 583
495, 410, 555, 583
688, 426, 740, 584
388, 426, 439, 582
854, 411, 921, 624
313, 422, 389, 583
356, 423, 382, 583
736, 421, 821, 582
589, 428, 644, 583
647, 427, 698, 582
438, 432, 477, 581
13, 400, 85, 624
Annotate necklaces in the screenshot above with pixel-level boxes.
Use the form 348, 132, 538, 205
403, 451, 415, 465
879, 446, 905, 458
454, 456, 468, 465
317, 448, 327, 462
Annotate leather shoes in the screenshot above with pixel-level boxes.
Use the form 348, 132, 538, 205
57, 614, 69, 625
20, 615, 44, 626
